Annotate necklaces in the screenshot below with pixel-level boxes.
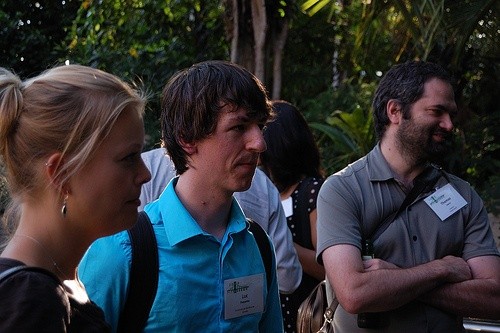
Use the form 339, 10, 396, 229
15, 231, 64, 274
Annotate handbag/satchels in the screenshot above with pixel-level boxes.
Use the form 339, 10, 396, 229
296, 281, 329, 333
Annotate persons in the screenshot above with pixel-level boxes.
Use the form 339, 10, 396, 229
0, 64, 153, 333
317, 60, 500, 333
136, 99, 326, 333
77, 60, 284, 333
